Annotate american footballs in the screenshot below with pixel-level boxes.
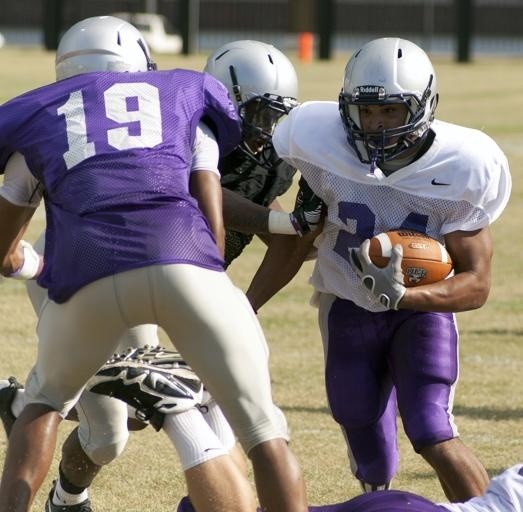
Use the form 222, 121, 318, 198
369, 230, 454, 286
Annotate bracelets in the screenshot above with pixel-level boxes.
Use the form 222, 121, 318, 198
13, 247, 40, 280
268, 210, 297, 234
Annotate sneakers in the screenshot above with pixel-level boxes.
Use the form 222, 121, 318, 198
87, 343, 203, 432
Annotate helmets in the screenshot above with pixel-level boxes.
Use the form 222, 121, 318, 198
55, 16, 158, 81
202, 40, 298, 153
338, 37, 439, 174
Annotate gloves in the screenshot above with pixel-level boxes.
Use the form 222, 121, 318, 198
293, 180, 324, 233
349, 239, 407, 311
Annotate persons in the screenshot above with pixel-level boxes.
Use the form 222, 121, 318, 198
84, 344, 523, 512
273, 37, 512, 504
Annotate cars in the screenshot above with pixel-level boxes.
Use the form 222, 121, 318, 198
110, 11, 185, 56
